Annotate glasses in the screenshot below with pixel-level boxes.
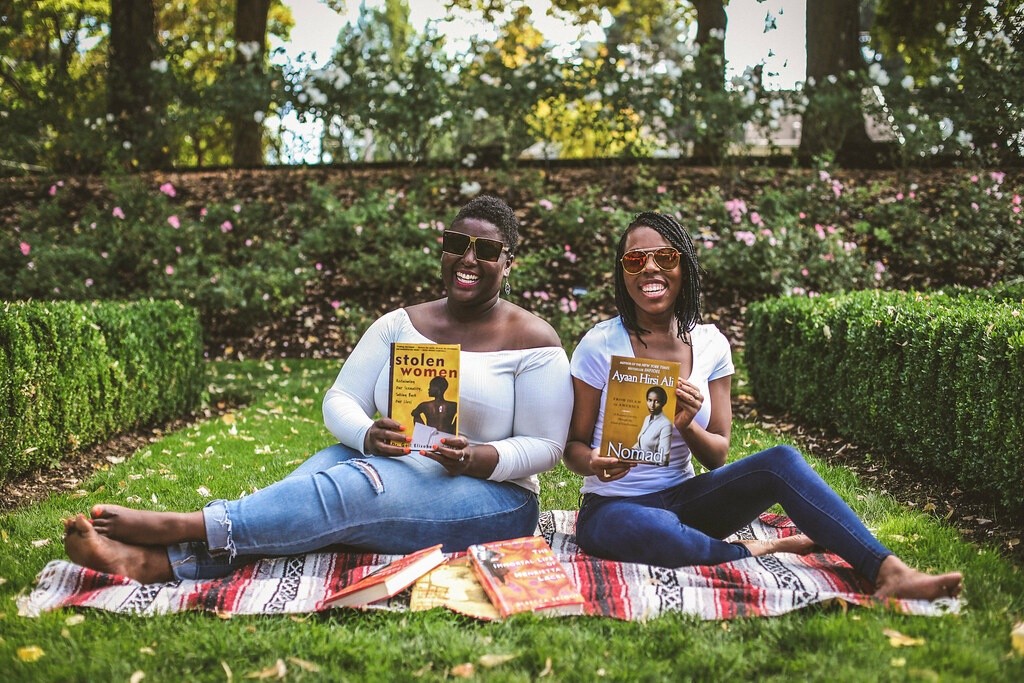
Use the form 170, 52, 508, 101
621, 246, 692, 275
442, 229, 512, 263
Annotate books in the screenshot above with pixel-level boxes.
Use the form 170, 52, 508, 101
602, 355, 681, 467
387, 341, 461, 451
325, 535, 585, 624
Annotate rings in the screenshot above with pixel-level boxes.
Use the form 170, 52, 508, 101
460, 451, 465, 460
603, 469, 611, 479
695, 391, 700, 398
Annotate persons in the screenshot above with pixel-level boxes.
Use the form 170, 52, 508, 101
411, 376, 458, 435
62, 197, 574, 584
623, 385, 674, 466
562, 212, 964, 602
